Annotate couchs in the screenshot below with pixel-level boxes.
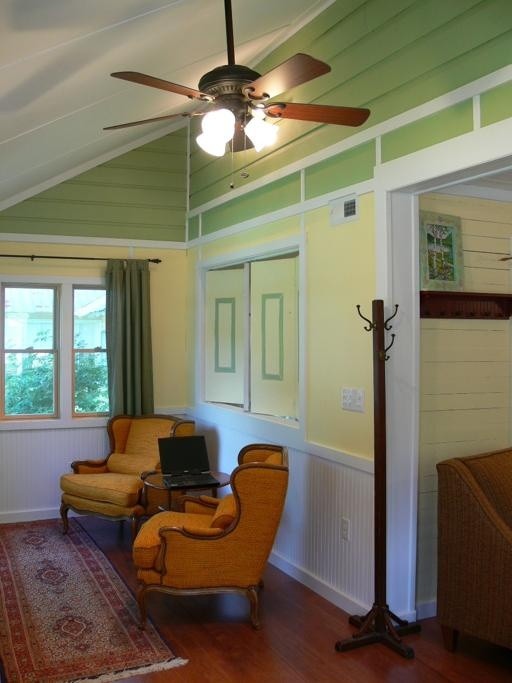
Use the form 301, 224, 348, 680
131, 443, 290, 631
435, 445, 512, 655
59, 413, 196, 555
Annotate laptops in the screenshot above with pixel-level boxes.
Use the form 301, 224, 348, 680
159, 435, 220, 489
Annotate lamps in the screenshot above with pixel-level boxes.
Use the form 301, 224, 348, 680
195, 133, 227, 158
243, 116, 280, 153
200, 107, 237, 144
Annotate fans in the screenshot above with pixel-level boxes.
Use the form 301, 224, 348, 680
103, 0, 372, 191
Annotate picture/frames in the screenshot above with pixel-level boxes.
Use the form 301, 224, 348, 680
418, 207, 465, 293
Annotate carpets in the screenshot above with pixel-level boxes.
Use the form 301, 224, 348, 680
0, 516, 190, 683
